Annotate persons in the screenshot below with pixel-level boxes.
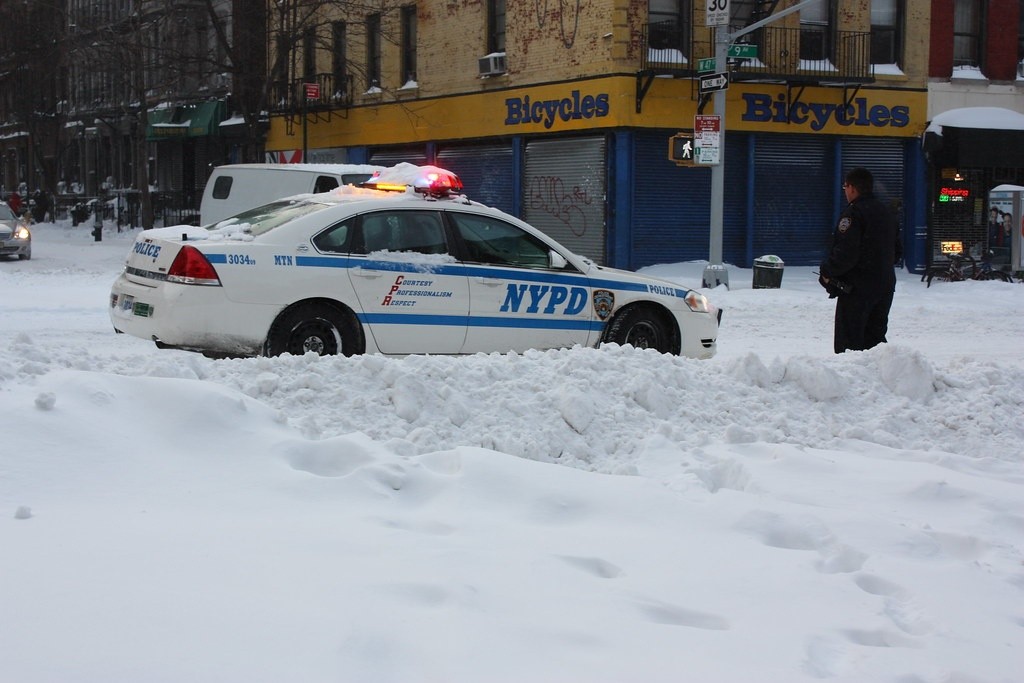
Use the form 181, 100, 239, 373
987, 207, 1012, 247
820, 169, 896, 354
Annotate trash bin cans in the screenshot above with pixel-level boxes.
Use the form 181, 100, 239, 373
751, 254, 784, 290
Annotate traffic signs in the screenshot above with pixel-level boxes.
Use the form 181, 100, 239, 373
699, 71, 729, 94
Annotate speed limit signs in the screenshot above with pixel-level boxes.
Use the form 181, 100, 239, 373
705, 0, 730, 27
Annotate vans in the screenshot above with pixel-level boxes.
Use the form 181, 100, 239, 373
199, 164, 389, 228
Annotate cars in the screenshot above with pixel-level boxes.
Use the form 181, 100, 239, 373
110, 166, 724, 363
0, 201, 33, 262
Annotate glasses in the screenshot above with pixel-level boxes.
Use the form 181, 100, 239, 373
842, 184, 850, 189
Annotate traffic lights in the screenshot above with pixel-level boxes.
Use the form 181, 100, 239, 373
669, 132, 712, 167
